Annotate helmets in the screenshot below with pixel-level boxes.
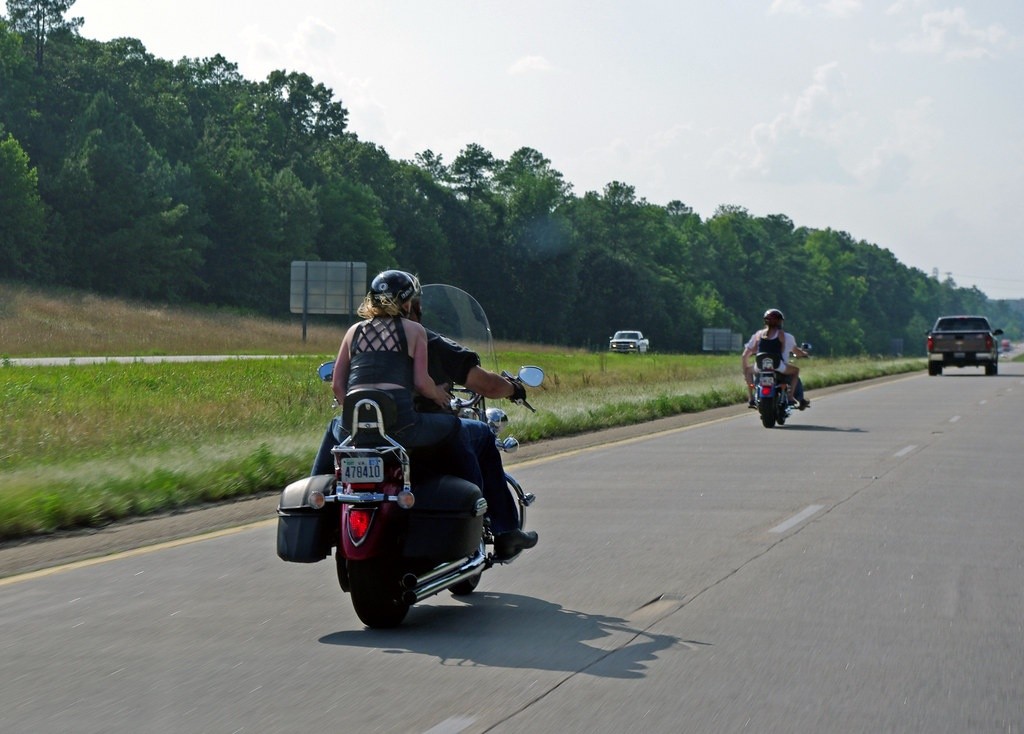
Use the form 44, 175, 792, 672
370, 269, 415, 307
404, 272, 423, 297
763, 309, 784, 325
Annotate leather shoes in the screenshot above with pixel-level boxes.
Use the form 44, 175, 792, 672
493, 528, 538, 560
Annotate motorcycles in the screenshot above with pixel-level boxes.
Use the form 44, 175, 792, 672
749, 343, 813, 429
275, 283, 547, 626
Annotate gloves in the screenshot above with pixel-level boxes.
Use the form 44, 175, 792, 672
507, 380, 526, 405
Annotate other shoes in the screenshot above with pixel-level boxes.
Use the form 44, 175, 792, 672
749, 400, 756, 405
799, 398, 810, 410
788, 398, 797, 405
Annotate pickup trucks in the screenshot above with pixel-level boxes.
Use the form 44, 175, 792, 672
609, 331, 649, 356
925, 316, 1005, 377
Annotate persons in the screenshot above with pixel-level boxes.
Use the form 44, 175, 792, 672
309, 269, 540, 562
740, 309, 810, 411
